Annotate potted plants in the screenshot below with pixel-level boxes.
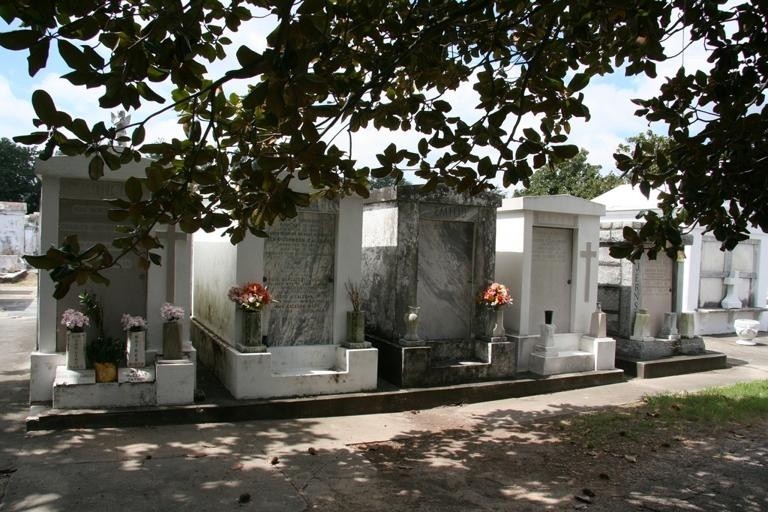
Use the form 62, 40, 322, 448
77, 287, 114, 363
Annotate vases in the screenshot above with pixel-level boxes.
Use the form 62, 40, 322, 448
124, 328, 147, 368
480, 303, 506, 338
65, 329, 87, 371
237, 306, 263, 347
398, 305, 423, 341
160, 316, 183, 361
345, 309, 367, 343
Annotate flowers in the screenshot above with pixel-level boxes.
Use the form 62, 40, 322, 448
59, 307, 91, 333
159, 301, 185, 322
474, 279, 514, 311
227, 280, 282, 314
118, 309, 150, 333
